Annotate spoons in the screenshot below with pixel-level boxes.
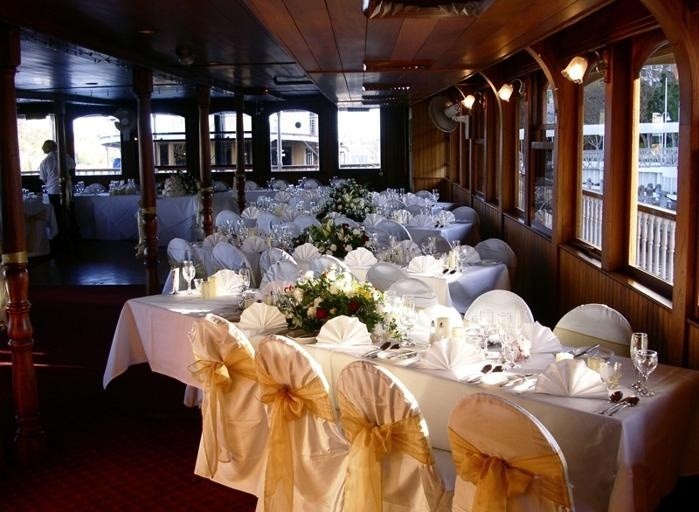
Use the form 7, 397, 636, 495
362, 341, 390, 358
609, 397, 639, 416
467, 364, 492, 383
599, 391, 623, 414
370, 343, 400, 358
602, 397, 631, 414
474, 365, 503, 385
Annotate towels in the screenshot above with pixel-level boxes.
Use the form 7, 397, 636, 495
240, 303, 286, 328
204, 234, 230, 247
317, 316, 370, 345
240, 237, 267, 253
395, 211, 412, 223
535, 359, 609, 400
208, 271, 242, 295
275, 192, 290, 202
516, 323, 563, 354
241, 207, 258, 219
363, 214, 384, 225
420, 306, 461, 331
407, 256, 442, 273
345, 248, 377, 265
420, 339, 482, 370
436, 211, 455, 223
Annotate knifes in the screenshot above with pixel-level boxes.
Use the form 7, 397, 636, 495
574, 344, 600, 358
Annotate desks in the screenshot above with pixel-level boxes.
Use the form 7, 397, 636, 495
407, 223, 474, 246
74, 193, 137, 241
214, 190, 276, 214
156, 197, 205, 247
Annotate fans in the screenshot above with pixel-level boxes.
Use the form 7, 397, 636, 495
428, 95, 473, 141
114, 108, 137, 143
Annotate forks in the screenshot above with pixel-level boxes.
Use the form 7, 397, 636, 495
495, 374, 522, 387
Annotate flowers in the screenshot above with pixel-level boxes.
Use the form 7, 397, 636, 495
277, 215, 386, 260
236, 264, 418, 343
315, 176, 379, 221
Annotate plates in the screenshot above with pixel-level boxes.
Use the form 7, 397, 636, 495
573, 346, 615, 358
287, 329, 320, 344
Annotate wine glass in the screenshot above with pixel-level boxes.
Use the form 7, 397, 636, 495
631, 333, 647, 388
239, 268, 251, 302
636, 350, 657, 397
433, 193, 439, 206
43, 179, 134, 195
497, 312, 511, 356
385, 291, 415, 340
182, 261, 195, 295
481, 310, 493, 355
371, 232, 467, 273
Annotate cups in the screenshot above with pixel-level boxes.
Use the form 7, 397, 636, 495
600, 360, 623, 388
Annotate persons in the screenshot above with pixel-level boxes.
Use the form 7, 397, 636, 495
40, 140, 76, 231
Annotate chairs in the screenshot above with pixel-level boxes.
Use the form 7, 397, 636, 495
103, 298, 699, 512
374, 219, 412, 240
256, 212, 280, 230
258, 249, 295, 263
417, 235, 452, 255
167, 239, 202, 277
186, 314, 255, 498
209, 242, 256, 289
332, 360, 446, 511
292, 214, 321, 228
258, 259, 298, 285
449, 394, 572, 512
449, 264, 509, 314
553, 303, 630, 345
364, 261, 406, 292
256, 334, 329, 510
451, 206, 480, 225
23, 197, 50, 254
462, 289, 534, 329
475, 238, 517, 264
309, 255, 342, 269
386, 276, 437, 310
215, 209, 242, 228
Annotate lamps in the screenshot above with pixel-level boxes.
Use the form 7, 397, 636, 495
460, 90, 487, 111
495, 77, 529, 102
560, 46, 612, 86
